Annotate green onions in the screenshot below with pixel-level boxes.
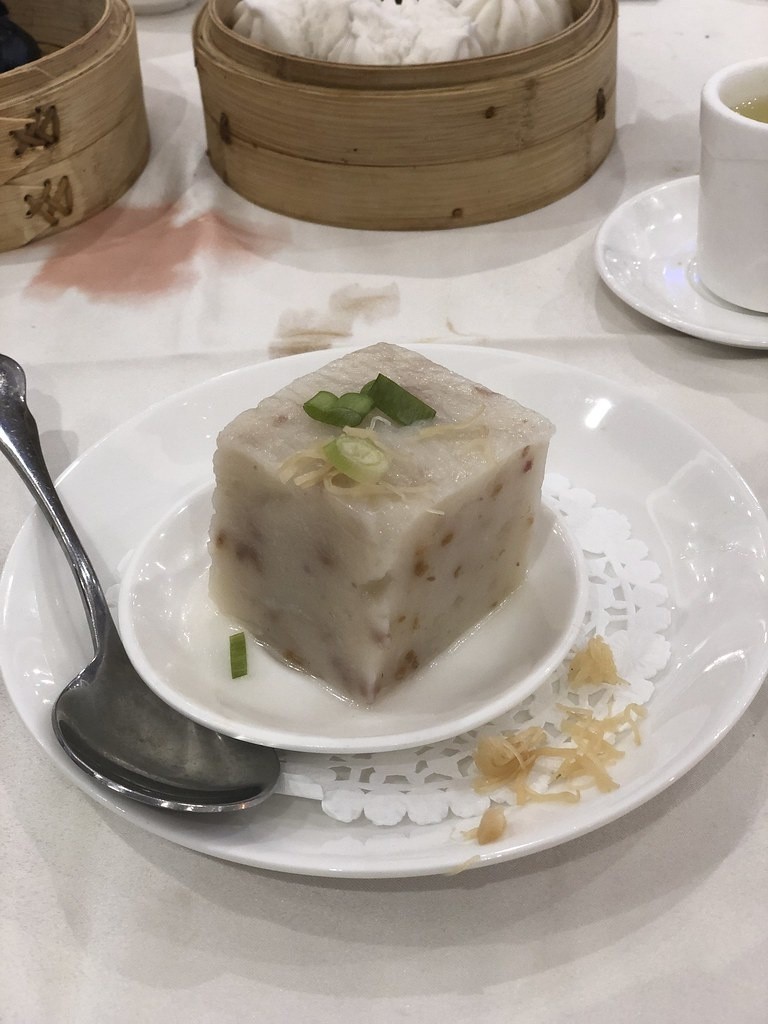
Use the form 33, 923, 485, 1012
229, 372, 440, 680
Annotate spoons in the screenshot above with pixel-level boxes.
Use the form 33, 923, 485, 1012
1, 353, 281, 815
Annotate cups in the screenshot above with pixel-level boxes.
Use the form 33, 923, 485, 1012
694, 55, 768, 314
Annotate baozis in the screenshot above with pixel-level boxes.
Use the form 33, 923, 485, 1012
227, 0, 573, 64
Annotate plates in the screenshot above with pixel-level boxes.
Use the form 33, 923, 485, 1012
592, 175, 768, 351
117, 478, 590, 754
1, 343, 767, 882
